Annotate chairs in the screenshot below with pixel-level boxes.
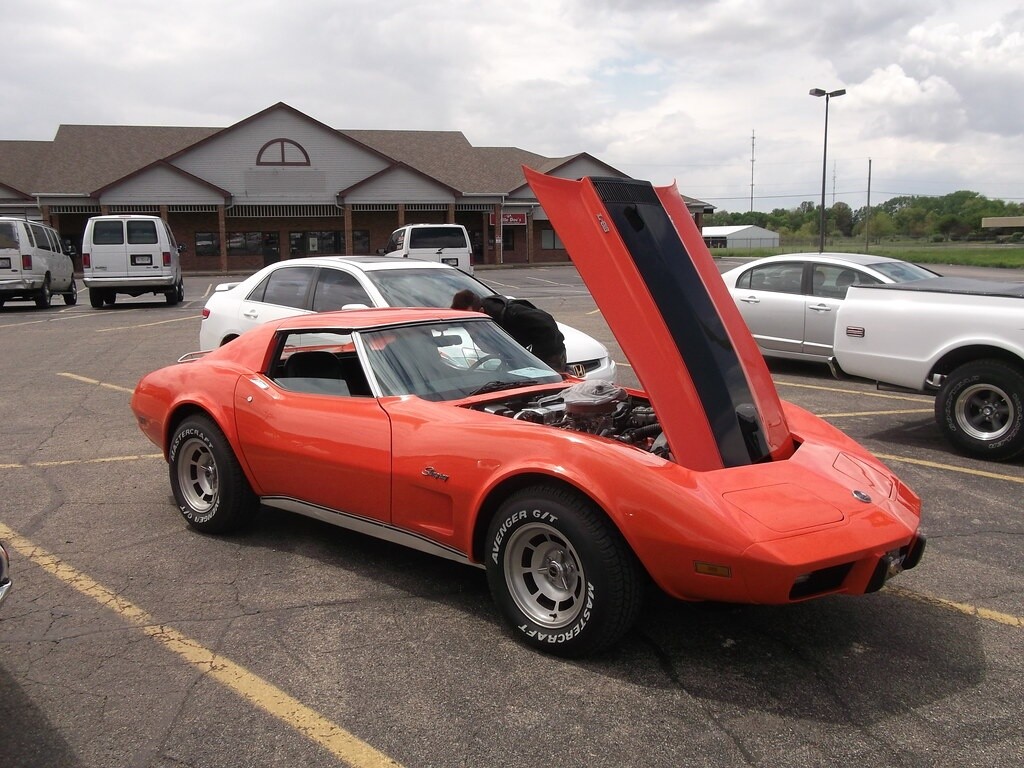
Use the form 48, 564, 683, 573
749, 271, 771, 290
813, 271, 826, 287
284, 351, 358, 395
382, 335, 459, 383
836, 271, 856, 286
778, 269, 800, 292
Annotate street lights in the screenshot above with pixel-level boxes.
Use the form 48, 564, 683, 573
809, 82, 847, 253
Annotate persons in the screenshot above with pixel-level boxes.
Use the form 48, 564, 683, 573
449, 288, 568, 378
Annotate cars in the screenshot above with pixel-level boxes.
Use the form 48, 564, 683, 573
722, 250, 944, 380
198, 255, 620, 384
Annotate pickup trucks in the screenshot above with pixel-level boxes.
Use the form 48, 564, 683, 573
827, 274, 1024, 463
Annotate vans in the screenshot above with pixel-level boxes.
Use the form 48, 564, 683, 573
81, 214, 187, 308
377, 223, 476, 280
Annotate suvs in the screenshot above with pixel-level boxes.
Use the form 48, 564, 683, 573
0, 217, 80, 311
127, 158, 928, 656
